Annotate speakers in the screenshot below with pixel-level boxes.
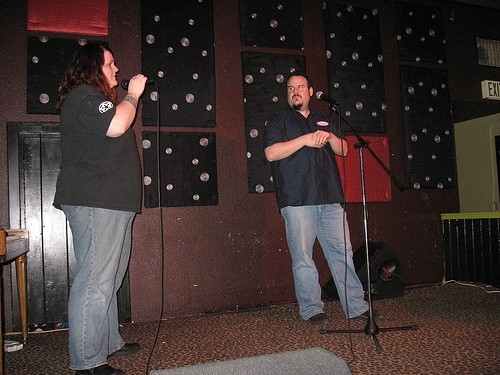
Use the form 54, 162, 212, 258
324, 241, 404, 301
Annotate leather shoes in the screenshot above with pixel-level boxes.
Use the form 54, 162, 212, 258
308, 313, 333, 325
357, 309, 385, 320
112, 342, 142, 355
75, 364, 127, 375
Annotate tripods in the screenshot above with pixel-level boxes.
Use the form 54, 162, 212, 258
320, 104, 418, 352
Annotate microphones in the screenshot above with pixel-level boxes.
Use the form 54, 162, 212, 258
121, 79, 154, 90
316, 91, 339, 106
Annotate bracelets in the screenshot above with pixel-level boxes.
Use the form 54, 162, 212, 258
327, 132, 334, 142
123, 95, 138, 109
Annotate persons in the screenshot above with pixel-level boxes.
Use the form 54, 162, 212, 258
53, 41, 148, 375
263, 71, 385, 325
